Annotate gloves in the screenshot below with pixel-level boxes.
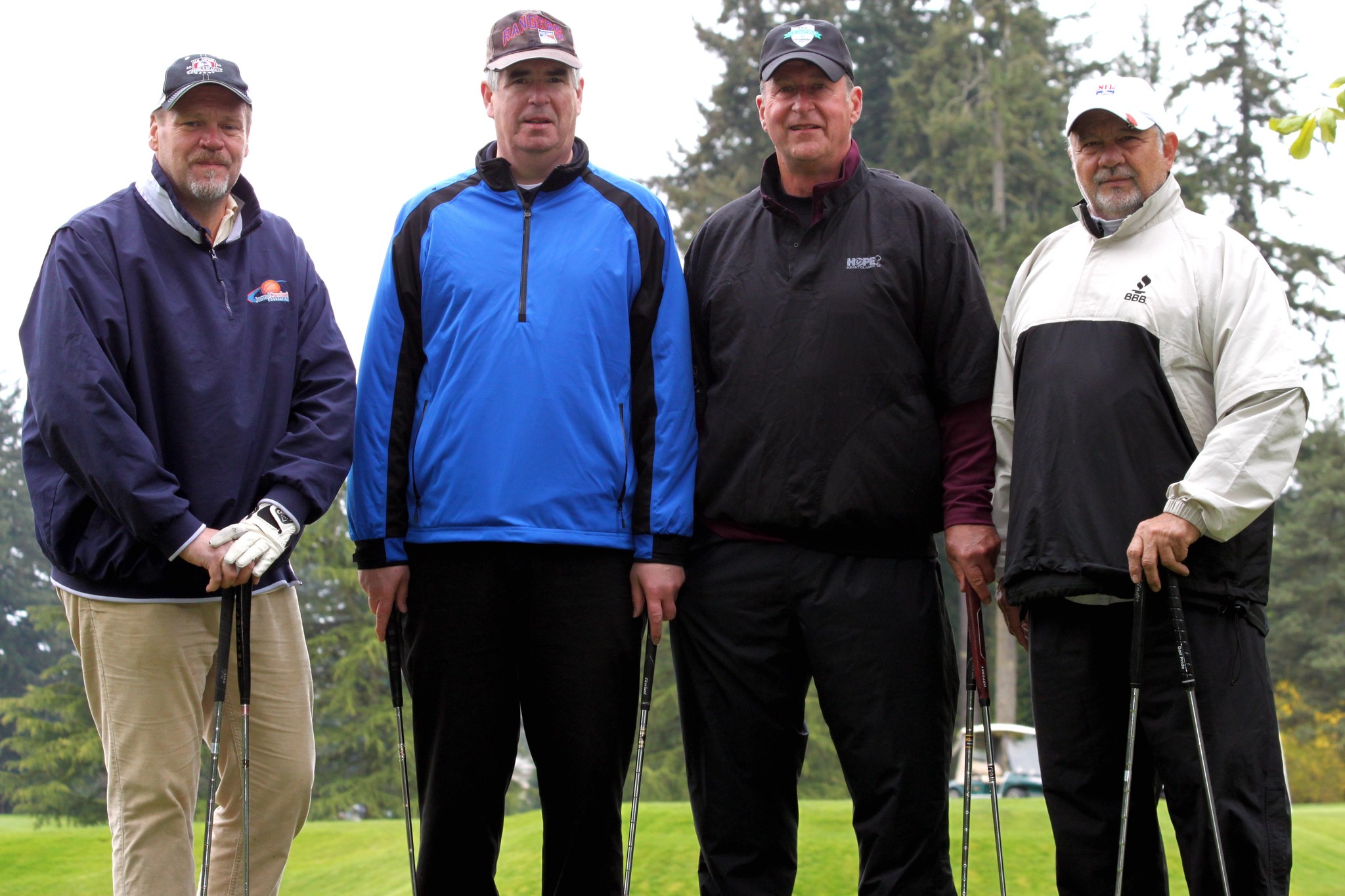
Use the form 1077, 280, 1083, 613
208, 502, 296, 578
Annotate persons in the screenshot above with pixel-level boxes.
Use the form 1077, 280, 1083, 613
10, 57, 358, 895
343, 9, 693, 895
990, 71, 1310, 896
664, 16, 1007, 895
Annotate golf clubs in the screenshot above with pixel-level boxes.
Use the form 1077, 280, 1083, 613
623, 616, 658, 896
234, 574, 254, 896
200, 586, 235, 896
1114, 570, 1146, 896
1162, 565, 1234, 896
961, 621, 977, 896
384, 599, 419, 896
964, 573, 1012, 896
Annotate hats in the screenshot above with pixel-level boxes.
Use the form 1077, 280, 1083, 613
150, 54, 254, 108
1066, 75, 1166, 136
484, 9, 582, 70
758, 18, 855, 82
